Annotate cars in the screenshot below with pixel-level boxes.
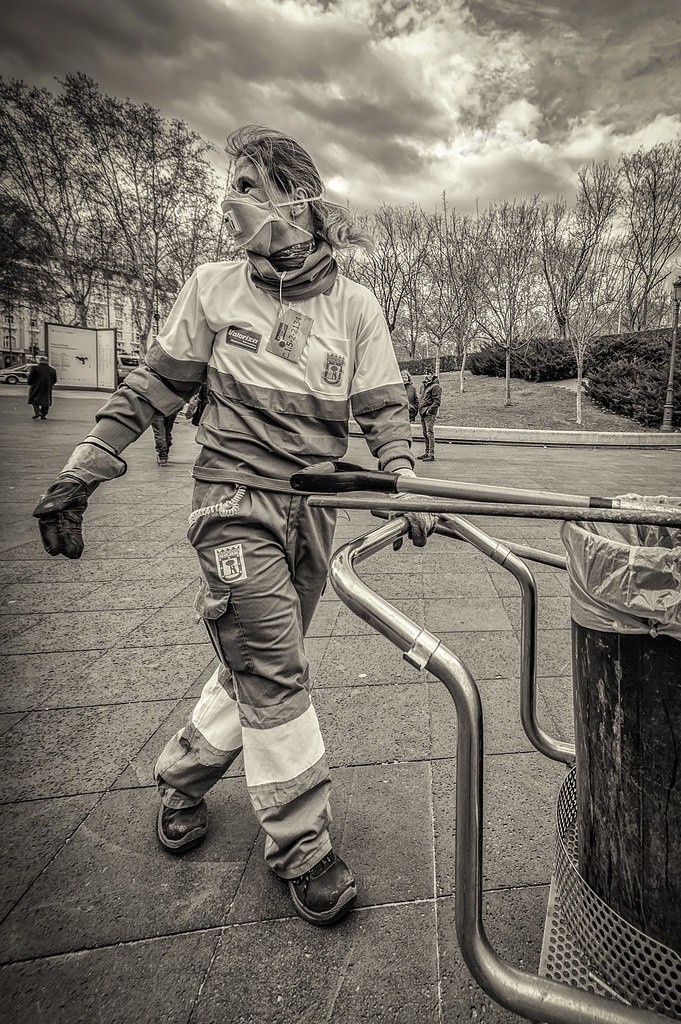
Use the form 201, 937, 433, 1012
117, 354, 141, 384
0, 363, 39, 384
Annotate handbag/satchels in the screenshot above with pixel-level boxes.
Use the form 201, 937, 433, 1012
184, 385, 202, 419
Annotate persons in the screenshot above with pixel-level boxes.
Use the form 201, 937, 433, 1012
34, 124, 443, 923
27, 356, 57, 419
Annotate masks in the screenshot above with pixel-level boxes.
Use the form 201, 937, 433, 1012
220, 188, 279, 257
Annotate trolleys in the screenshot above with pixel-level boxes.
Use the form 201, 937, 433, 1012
328, 502, 681, 1024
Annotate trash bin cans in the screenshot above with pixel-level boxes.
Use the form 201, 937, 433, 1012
562, 492, 681, 958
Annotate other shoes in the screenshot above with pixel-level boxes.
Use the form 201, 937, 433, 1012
417, 454, 435, 461
156, 773, 208, 854
287, 849, 356, 926
32, 412, 47, 420
156, 454, 167, 467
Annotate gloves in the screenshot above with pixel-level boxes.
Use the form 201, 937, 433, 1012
32, 441, 128, 560
388, 468, 440, 551
